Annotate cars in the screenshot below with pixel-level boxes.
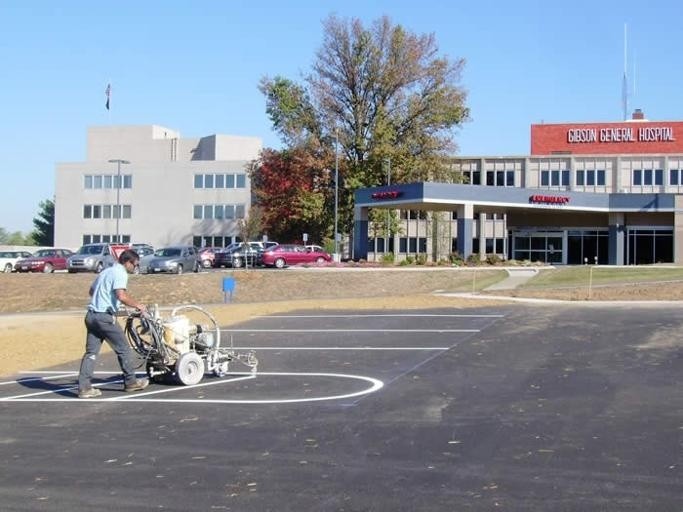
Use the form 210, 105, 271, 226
199, 242, 278, 268
14, 249, 74, 273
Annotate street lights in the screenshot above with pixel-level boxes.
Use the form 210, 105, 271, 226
108, 159, 131, 243
333, 126, 341, 254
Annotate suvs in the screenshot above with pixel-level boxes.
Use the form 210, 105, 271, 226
67, 244, 154, 275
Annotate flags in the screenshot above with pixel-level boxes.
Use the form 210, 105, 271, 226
105, 84, 110, 109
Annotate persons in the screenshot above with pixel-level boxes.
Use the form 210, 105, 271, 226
79, 249, 150, 399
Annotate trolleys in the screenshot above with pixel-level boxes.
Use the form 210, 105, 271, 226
119, 302, 259, 386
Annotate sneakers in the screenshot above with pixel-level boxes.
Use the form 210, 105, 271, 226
124, 378, 149, 392
78, 387, 102, 399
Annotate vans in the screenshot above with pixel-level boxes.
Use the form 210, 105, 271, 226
262, 244, 333, 268
0, 251, 32, 273
151, 247, 197, 274
